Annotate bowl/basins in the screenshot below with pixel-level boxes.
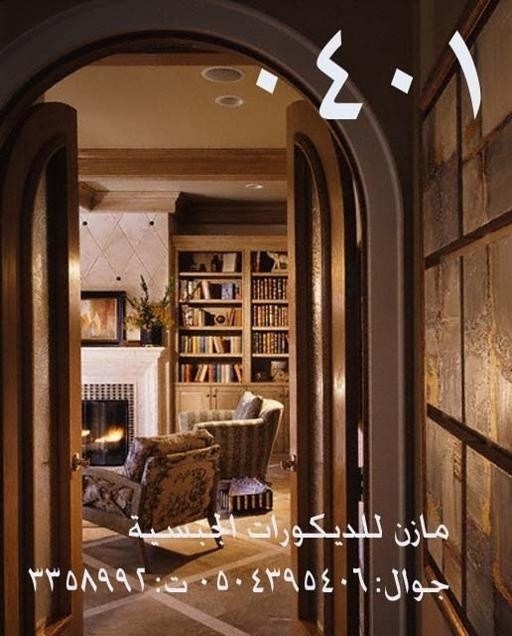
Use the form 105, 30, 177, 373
270, 360, 287, 370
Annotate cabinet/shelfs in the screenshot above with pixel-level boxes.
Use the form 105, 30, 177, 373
170, 234, 291, 460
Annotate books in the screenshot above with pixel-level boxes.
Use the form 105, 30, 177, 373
178, 250, 290, 383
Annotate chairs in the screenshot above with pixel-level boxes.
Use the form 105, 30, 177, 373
79, 388, 284, 574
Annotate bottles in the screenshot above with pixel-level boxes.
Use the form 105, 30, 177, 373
211, 252, 221, 272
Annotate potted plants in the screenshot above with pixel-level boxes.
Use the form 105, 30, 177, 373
120, 274, 179, 345
